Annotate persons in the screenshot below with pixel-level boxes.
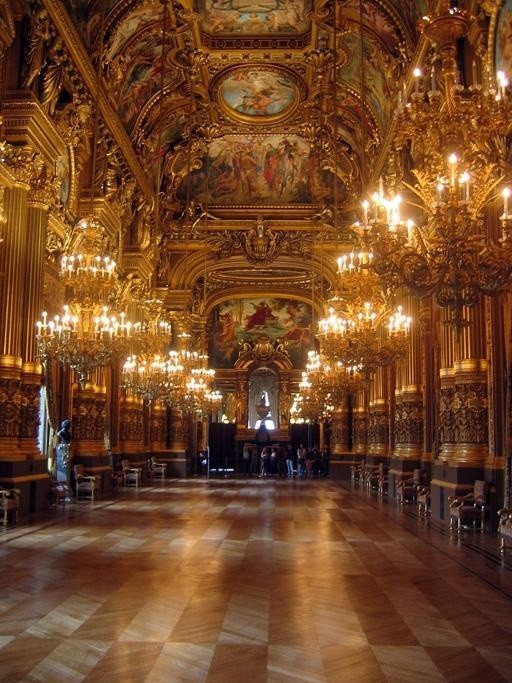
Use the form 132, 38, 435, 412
21, 8, 68, 119
243, 303, 271, 331
208, 1, 301, 33
57, 419, 71, 444
242, 443, 330, 479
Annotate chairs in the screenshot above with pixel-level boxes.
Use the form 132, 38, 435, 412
0, 451, 171, 531
348, 460, 511, 580
